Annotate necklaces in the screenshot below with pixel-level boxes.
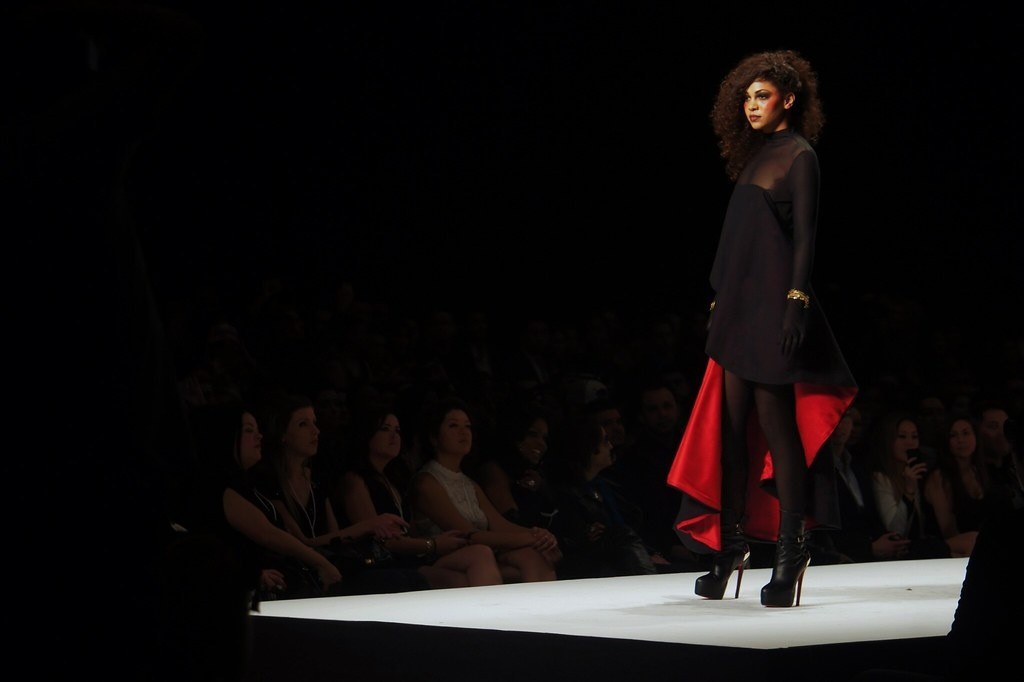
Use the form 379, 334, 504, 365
287, 479, 318, 539
384, 477, 407, 533
254, 487, 277, 521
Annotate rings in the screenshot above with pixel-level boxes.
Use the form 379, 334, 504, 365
551, 533, 555, 538
589, 526, 596, 532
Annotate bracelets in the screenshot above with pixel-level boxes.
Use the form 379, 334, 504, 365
710, 302, 716, 309
788, 289, 810, 308
417, 537, 435, 558
906, 489, 916, 496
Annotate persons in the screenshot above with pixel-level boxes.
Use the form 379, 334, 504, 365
167, 295, 1024, 598
665, 49, 860, 608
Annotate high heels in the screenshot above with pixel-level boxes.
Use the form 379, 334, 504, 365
761, 536, 811, 609
695, 533, 750, 600
